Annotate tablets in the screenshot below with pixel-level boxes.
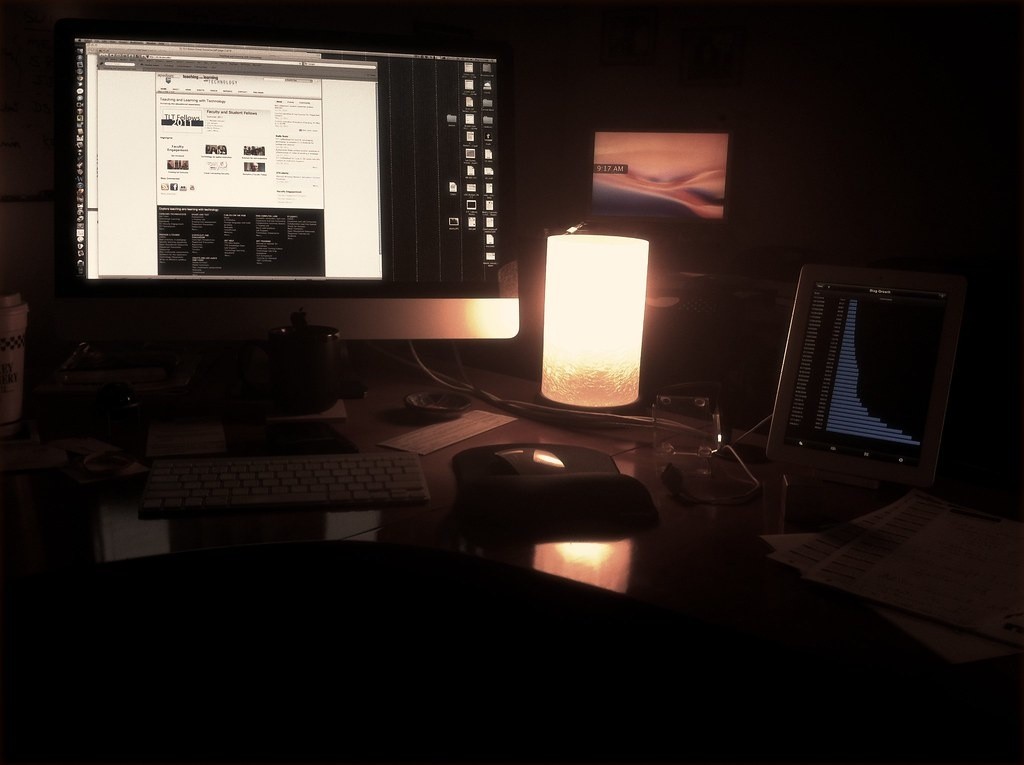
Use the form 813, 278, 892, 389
761, 263, 967, 488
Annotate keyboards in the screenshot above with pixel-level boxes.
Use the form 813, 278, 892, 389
138, 452, 431, 519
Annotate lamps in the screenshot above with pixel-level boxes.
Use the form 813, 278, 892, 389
541, 234, 649, 409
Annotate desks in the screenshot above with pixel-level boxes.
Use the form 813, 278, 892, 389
0, 361, 1024, 702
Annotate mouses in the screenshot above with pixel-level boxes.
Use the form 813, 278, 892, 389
495, 449, 566, 477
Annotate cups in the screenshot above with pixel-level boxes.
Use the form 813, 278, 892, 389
265, 326, 346, 413
0, 290, 31, 438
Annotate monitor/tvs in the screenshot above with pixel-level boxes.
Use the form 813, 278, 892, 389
584, 128, 733, 225
49, 19, 521, 422
